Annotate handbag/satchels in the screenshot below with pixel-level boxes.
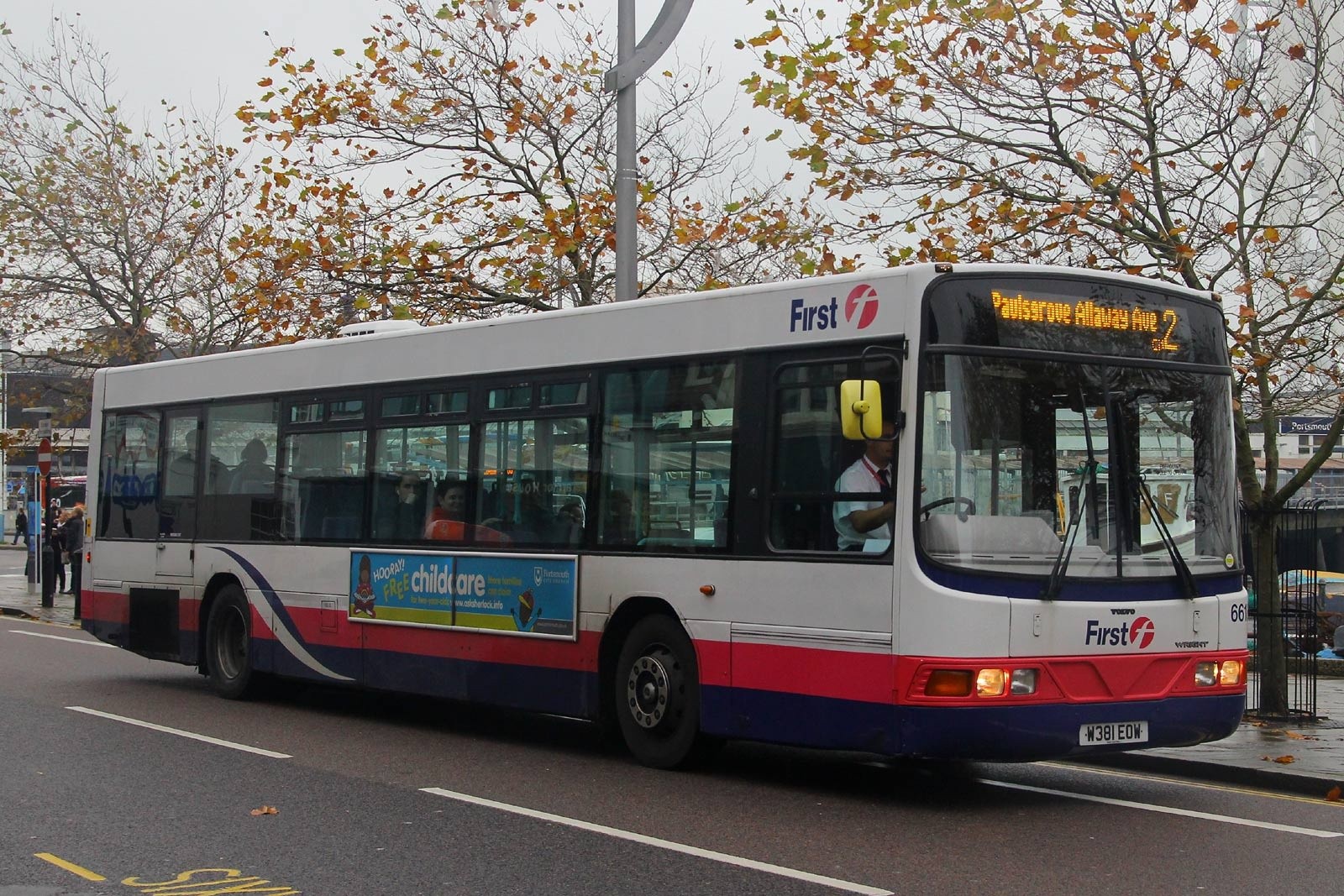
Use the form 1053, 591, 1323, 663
61, 551, 72, 564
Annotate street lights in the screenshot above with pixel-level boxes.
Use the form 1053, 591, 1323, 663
22, 406, 60, 609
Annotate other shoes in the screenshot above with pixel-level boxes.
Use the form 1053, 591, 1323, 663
65, 589, 74, 594
59, 587, 64, 593
11, 543, 15, 545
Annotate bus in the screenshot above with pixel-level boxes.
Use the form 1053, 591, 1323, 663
345, 430, 537, 490
81, 259, 1250, 775
39, 477, 89, 550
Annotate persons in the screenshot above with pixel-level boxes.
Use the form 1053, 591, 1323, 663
155, 429, 275, 541
377, 470, 640, 551
832, 415, 926, 552
10, 499, 84, 595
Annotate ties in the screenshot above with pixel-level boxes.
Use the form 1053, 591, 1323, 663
878, 469, 892, 532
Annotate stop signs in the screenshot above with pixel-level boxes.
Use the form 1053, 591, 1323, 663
37, 437, 52, 479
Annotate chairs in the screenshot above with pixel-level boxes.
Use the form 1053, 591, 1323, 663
108, 464, 540, 539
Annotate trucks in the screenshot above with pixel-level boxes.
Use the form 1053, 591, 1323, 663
1060, 474, 1196, 553
